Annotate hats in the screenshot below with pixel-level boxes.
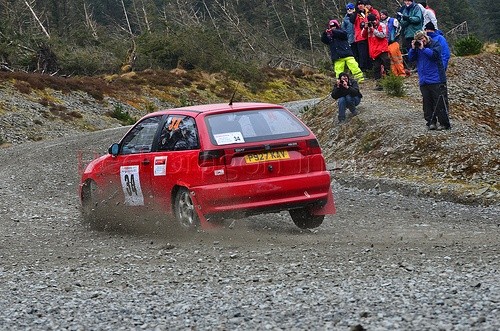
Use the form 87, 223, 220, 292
425, 22, 435, 30
380, 9, 388, 16
346, 3, 355, 8
356, 0, 364, 6
414, 30, 425, 39
339, 72, 349, 80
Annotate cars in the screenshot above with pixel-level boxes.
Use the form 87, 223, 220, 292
78, 101, 337, 236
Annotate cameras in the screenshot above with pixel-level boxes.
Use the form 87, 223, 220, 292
360, 22, 365, 28
414, 41, 420, 47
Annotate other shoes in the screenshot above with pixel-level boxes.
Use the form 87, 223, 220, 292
437, 122, 447, 131
429, 124, 437, 130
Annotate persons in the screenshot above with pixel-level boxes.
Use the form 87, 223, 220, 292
320, 19, 365, 88
340, 0, 438, 79
408, 29, 450, 131
332, 72, 362, 123
423, 21, 450, 114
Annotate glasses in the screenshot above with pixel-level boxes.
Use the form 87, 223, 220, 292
346, 8, 353, 10
